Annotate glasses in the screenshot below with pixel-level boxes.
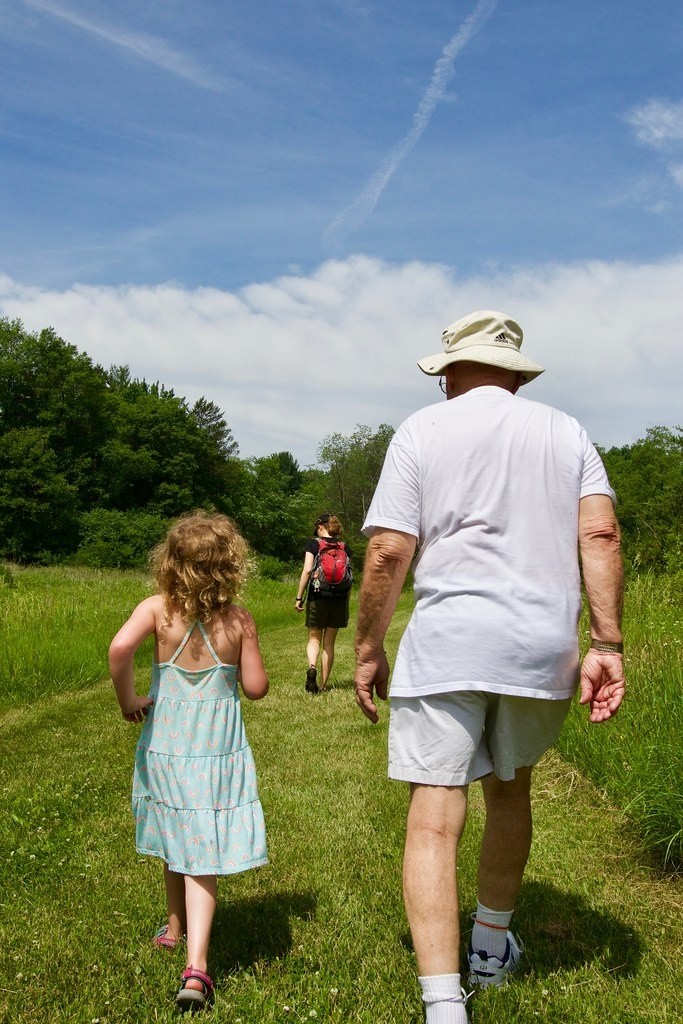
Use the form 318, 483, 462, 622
438, 370, 447, 394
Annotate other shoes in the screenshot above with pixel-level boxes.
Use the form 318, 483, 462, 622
306, 667, 318, 693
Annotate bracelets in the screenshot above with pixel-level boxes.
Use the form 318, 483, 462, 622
590, 639, 623, 654
296, 598, 302, 601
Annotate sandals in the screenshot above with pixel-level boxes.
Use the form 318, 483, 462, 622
176, 968, 214, 1010
155, 925, 184, 959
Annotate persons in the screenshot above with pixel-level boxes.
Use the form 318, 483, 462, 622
109, 509, 269, 1006
294, 515, 353, 695
353, 309, 626, 1024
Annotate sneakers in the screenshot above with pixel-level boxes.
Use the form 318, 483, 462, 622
468, 930, 519, 989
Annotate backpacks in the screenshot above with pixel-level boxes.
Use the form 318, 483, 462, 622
312, 538, 352, 593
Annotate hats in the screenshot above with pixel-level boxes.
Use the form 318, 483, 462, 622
314, 515, 331, 536
417, 311, 545, 387
